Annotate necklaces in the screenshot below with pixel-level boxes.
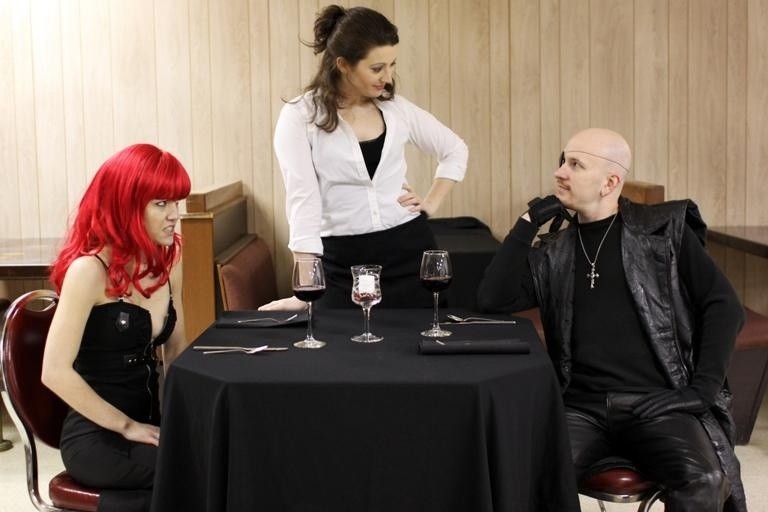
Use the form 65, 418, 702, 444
577, 211, 618, 288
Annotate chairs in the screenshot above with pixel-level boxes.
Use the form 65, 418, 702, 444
215, 229, 278, 311
579, 467, 668, 512
0, 289, 105, 512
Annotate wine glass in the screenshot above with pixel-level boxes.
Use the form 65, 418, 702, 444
419, 251, 453, 337
291, 258, 327, 349
350, 265, 384, 343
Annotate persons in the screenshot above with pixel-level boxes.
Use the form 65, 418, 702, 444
258, 4, 468, 311
41, 143, 191, 490
475, 126, 748, 511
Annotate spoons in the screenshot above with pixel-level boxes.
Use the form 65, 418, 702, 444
447, 314, 493, 322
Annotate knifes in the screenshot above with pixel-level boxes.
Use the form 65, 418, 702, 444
430, 320, 519, 325
193, 345, 289, 353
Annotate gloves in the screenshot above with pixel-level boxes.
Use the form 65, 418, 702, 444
631, 387, 712, 419
529, 194, 572, 233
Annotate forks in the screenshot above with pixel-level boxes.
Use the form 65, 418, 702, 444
237, 313, 299, 324
202, 345, 268, 355
